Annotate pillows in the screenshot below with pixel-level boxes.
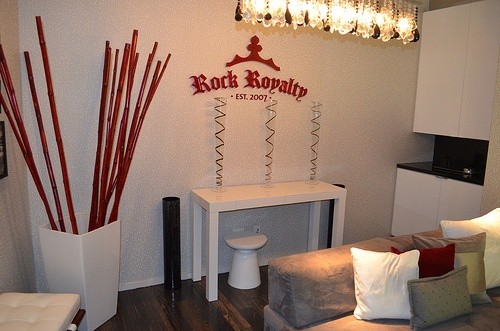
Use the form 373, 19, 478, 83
412, 233, 491, 305
440, 207, 500, 289
392, 243, 455, 278
349, 247, 421, 321
408, 267, 473, 331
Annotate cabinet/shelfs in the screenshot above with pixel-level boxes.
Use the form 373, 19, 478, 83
390, 166, 483, 237
412, 1, 499, 141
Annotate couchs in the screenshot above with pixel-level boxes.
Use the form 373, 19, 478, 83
264, 230, 500, 331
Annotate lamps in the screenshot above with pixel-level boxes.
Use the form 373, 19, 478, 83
234, 0, 421, 44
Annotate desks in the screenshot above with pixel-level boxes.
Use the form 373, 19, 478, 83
190, 180, 347, 302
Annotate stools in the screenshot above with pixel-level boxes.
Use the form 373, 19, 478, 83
1, 292, 86, 331
224, 231, 267, 290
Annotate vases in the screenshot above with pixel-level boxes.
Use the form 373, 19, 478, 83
39, 217, 121, 331
